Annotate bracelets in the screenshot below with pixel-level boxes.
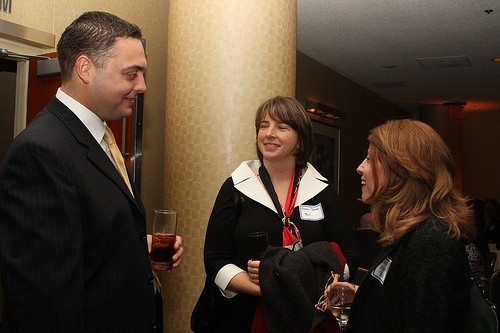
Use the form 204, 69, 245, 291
349, 282, 355, 288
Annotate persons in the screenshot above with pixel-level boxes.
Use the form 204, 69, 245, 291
190, 95, 359, 333
0, 11, 183, 333
324, 119, 500, 333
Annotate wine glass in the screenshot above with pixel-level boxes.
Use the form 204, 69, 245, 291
324, 273, 358, 333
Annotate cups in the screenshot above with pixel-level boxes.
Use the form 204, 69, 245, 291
150, 209, 178, 274
246, 230, 269, 261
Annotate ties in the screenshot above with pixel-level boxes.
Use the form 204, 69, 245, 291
103, 125, 135, 198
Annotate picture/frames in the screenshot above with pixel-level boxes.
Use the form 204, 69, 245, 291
308, 119, 342, 196
